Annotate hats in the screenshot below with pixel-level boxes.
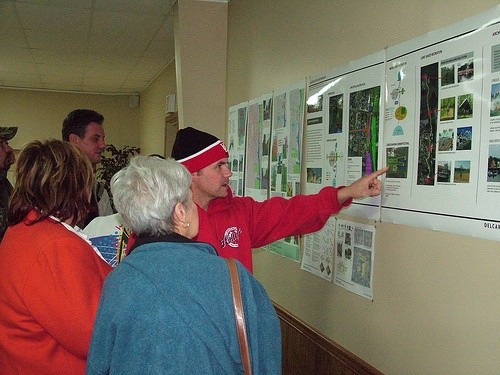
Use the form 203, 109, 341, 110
171, 127, 229, 173
0, 126, 18, 141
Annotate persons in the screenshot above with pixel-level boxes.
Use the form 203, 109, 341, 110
62, 108, 105, 230
86, 155, 282, 375
0, 126, 18, 244
0, 138, 115, 375
126, 127, 390, 276
